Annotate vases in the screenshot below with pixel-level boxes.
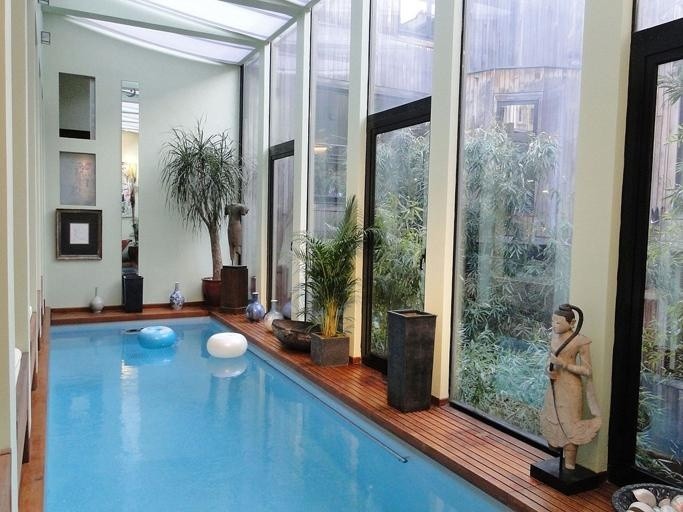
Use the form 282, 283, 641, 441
169, 282, 185, 311
246, 291, 265, 322
90, 287, 105, 313
264, 300, 285, 333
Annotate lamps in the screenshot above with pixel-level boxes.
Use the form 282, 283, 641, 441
41, 31, 50, 45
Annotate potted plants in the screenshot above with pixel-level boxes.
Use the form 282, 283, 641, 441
127, 174, 138, 264
289, 193, 389, 368
154, 115, 253, 310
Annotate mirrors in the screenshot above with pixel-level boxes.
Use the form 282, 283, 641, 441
121, 81, 140, 275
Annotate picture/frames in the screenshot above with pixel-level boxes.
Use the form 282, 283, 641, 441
55, 208, 103, 261
